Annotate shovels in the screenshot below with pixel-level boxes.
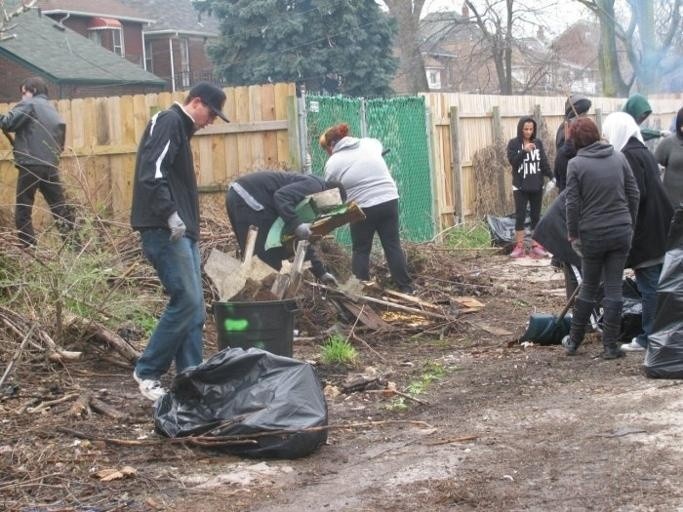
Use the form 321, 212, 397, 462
506, 280, 582, 347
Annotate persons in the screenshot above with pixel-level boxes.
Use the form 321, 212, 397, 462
319, 123, 417, 292
505, 115, 556, 260
224, 170, 348, 290
529, 93, 683, 361
129, 80, 231, 403
0, 77, 82, 253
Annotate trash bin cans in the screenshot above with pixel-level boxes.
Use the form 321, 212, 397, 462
211, 299, 299, 358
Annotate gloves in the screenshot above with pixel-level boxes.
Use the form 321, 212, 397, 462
320, 271, 339, 288
167, 210, 186, 244
295, 222, 314, 240
571, 237, 584, 257
660, 129, 672, 137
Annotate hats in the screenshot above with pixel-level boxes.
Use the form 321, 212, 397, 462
190, 80, 230, 122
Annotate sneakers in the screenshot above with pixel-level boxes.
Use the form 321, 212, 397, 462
623, 338, 644, 351
509, 246, 527, 257
531, 245, 548, 258
403, 289, 418, 296
132, 367, 167, 403
561, 335, 577, 356
604, 344, 624, 359
